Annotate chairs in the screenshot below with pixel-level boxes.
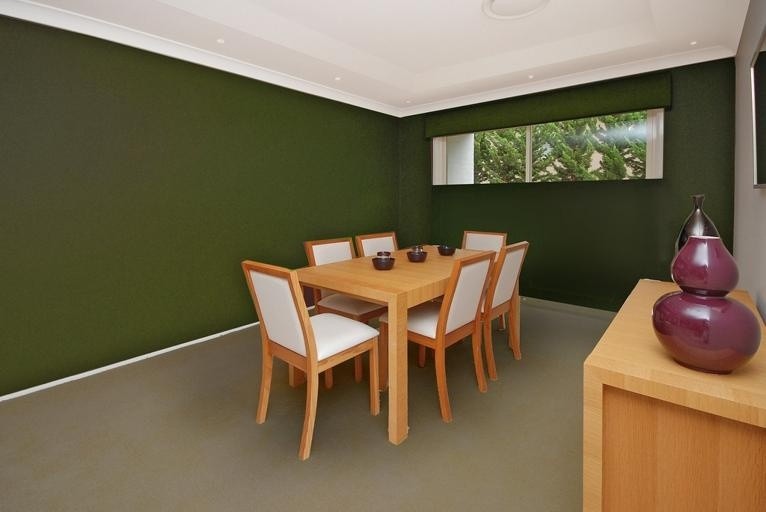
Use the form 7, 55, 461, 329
242, 259, 381, 458
431, 232, 507, 331
428, 240, 529, 382
380, 249, 497, 424
303, 237, 394, 382
355, 233, 400, 258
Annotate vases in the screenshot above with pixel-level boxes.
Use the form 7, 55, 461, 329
652, 195, 761, 375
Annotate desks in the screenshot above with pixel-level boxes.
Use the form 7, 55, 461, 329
292, 244, 500, 447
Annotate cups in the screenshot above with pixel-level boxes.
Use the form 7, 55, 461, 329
413, 246, 423, 253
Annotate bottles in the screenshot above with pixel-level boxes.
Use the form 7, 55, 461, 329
377, 252, 391, 258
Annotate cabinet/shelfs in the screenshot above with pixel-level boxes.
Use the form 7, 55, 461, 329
582, 278, 766, 512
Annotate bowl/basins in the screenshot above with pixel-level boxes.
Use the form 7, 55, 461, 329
407, 251, 427, 262
372, 258, 394, 270
438, 244, 455, 256
